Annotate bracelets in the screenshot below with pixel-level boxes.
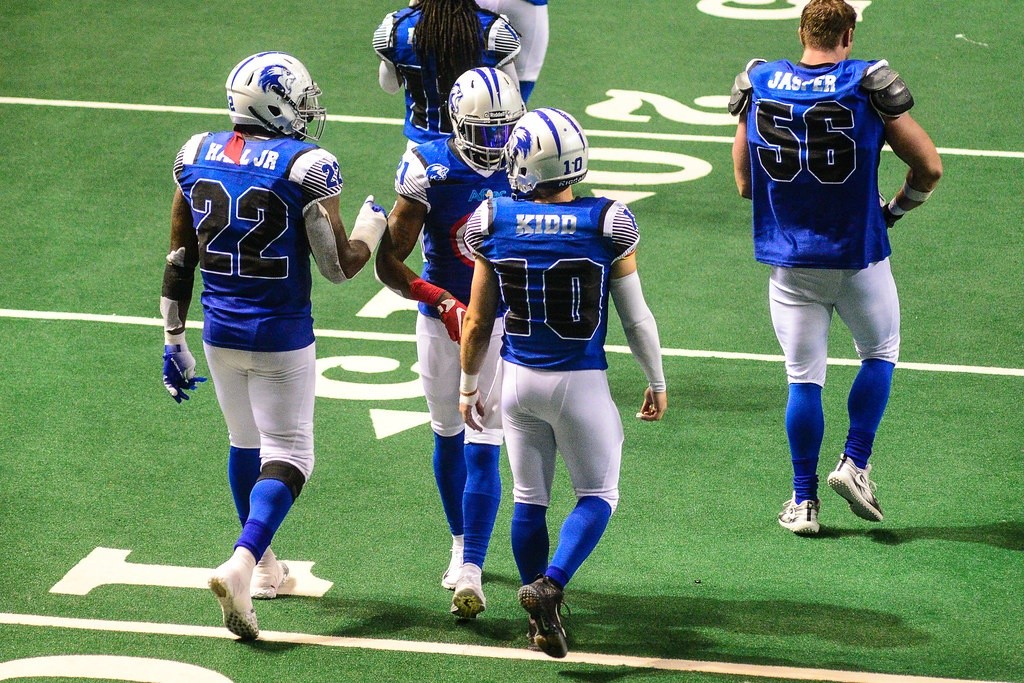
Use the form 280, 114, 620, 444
888, 197, 907, 217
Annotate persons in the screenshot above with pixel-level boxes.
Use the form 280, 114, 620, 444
458, 108, 668, 658
372, 0, 550, 154
728, 0, 942, 534
374, 68, 527, 618
159, 51, 389, 641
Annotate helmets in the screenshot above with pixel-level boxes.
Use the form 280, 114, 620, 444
503, 107, 589, 200
448, 68, 525, 172
224, 52, 327, 141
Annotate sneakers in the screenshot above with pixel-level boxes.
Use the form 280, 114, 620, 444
250, 562, 290, 599
776, 491, 820, 536
450, 563, 486, 619
517, 573, 571, 659
208, 554, 259, 639
442, 545, 471, 589
828, 453, 884, 523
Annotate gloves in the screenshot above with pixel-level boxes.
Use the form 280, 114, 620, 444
436, 296, 468, 345
161, 338, 208, 403
349, 195, 388, 258
